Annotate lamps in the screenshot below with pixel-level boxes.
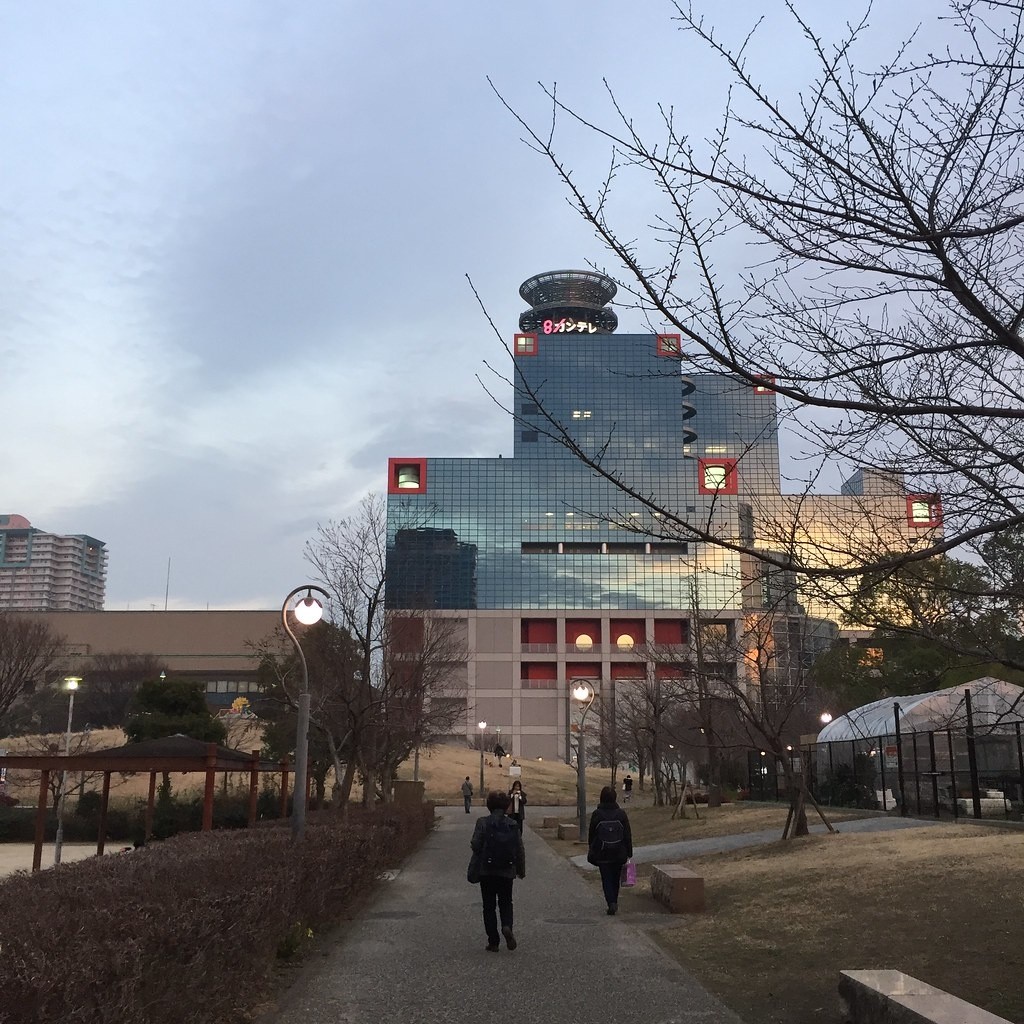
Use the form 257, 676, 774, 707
281, 585, 332, 835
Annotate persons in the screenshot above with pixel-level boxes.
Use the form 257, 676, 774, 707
508, 780, 527, 836
587, 786, 634, 915
495, 743, 505, 768
471, 791, 526, 952
461, 777, 473, 814
623, 775, 633, 803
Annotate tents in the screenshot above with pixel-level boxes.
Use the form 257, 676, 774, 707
818, 677, 1024, 792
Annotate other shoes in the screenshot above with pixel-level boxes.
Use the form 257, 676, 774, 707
501, 926, 517, 950
485, 942, 500, 952
499, 764, 502, 767
607, 903, 618, 915
624, 799, 626, 803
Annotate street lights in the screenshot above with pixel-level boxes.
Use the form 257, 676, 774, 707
55, 674, 83, 864
571, 678, 596, 842
478, 719, 488, 797
281, 585, 330, 840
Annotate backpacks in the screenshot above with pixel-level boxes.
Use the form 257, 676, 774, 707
591, 809, 625, 859
480, 815, 517, 869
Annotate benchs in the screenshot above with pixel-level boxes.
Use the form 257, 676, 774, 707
559, 823, 579, 841
838, 969, 1016, 1024
543, 815, 558, 829
652, 864, 704, 913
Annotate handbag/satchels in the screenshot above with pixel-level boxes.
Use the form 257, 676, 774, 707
622, 784, 625, 790
471, 792, 473, 796
621, 859, 636, 887
504, 801, 512, 815
499, 751, 503, 756
